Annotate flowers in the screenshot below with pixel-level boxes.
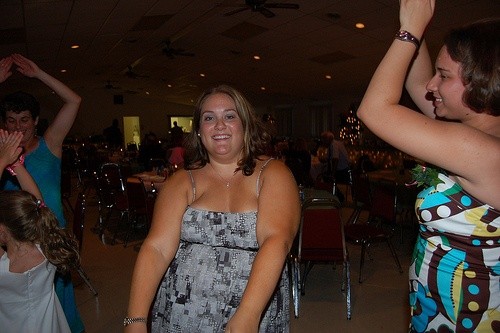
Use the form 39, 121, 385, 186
405, 158, 448, 191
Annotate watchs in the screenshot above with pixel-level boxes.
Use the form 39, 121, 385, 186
125, 316, 147, 326
394, 30, 420, 49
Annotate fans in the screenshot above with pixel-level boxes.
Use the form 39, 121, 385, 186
93, 80, 123, 91
118, 65, 150, 80
151, 39, 196, 59
217, 0, 299, 18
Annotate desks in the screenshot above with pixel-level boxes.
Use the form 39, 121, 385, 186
298, 189, 339, 209
126, 170, 165, 197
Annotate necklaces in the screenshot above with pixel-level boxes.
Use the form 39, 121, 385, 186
209, 162, 238, 189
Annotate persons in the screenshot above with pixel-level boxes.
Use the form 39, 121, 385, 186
123, 83, 303, 333
263, 126, 418, 204
0, 52, 199, 333
357, 0, 500, 333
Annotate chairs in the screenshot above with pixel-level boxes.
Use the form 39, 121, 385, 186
61, 135, 423, 320
